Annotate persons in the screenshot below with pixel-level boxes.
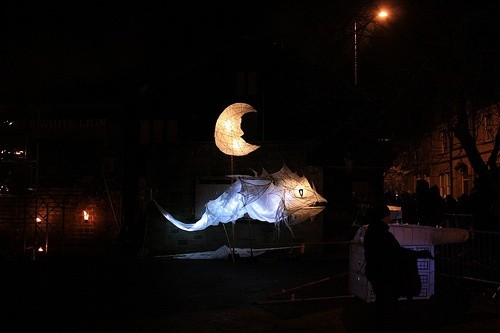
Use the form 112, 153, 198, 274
361, 199, 422, 333
399, 180, 500, 231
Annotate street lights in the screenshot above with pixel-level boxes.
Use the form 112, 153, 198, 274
354, 3, 392, 90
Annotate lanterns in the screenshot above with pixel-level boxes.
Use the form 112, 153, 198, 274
214, 102, 260, 156
154, 163, 328, 232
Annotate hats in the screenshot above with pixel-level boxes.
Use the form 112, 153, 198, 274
372, 202, 391, 220
